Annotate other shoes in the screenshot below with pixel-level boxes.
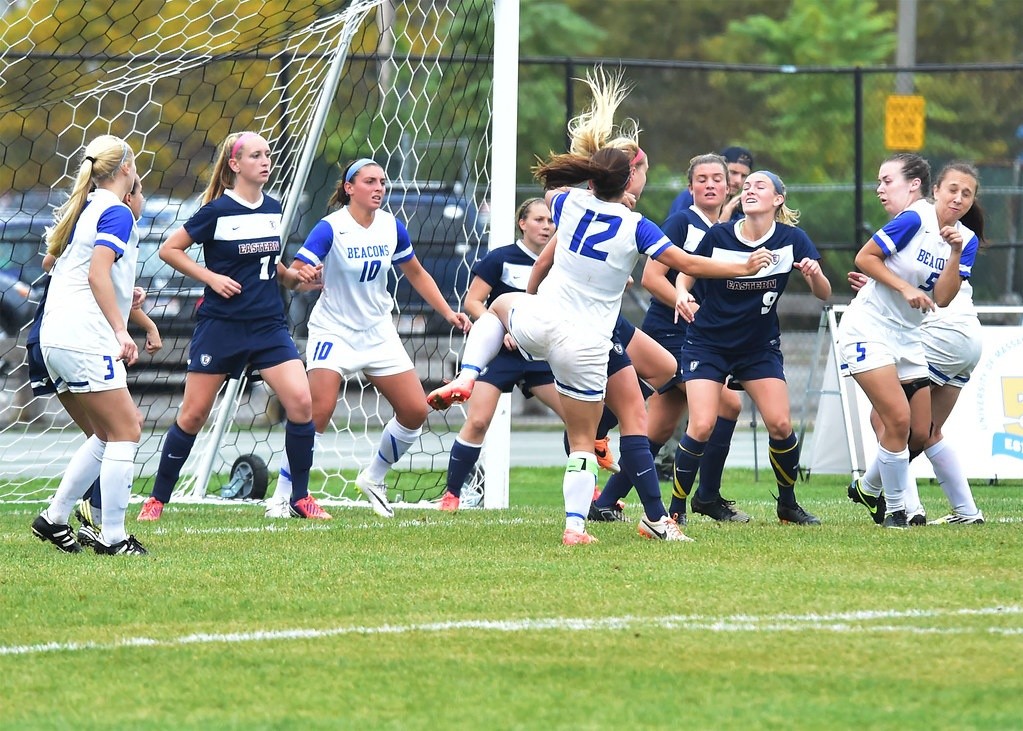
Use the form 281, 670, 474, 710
654, 444, 674, 481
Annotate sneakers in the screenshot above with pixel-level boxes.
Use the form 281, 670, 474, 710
30, 509, 83, 554
690, 489, 749, 522
847, 479, 887, 524
882, 510, 908, 530
777, 503, 821, 525
668, 511, 687, 526
594, 436, 621, 473
927, 509, 985, 525
354, 469, 395, 518
638, 513, 696, 543
289, 494, 332, 520
265, 496, 291, 518
426, 379, 475, 411
439, 491, 460, 511
94, 532, 149, 556
137, 497, 164, 521
563, 529, 600, 544
907, 505, 926, 526
77, 524, 102, 547
591, 485, 625, 508
587, 500, 632, 523
74, 497, 94, 528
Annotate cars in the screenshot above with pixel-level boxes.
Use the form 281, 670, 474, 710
0, 192, 491, 428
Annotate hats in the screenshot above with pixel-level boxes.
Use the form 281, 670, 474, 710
721, 148, 753, 170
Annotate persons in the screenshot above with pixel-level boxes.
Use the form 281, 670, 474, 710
39, 134, 147, 556
265, 156, 472, 521
426, 68, 835, 544
845, 153, 986, 528
136, 129, 335, 524
27, 172, 161, 546
834, 162, 985, 534
